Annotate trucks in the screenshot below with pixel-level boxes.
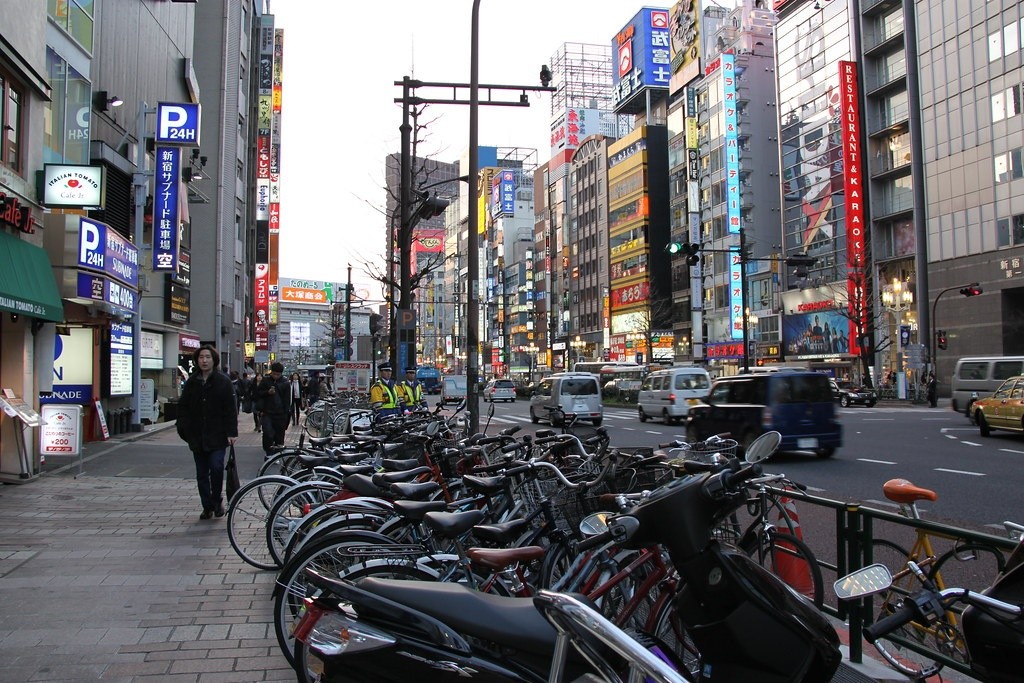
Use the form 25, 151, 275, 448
416, 366, 441, 395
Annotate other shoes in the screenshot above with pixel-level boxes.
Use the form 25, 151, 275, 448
200, 505, 213, 520
254, 425, 262, 433
292, 421, 295, 426
297, 420, 299, 426
213, 502, 225, 517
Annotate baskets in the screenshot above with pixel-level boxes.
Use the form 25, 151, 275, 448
601, 447, 655, 491
683, 439, 738, 463
631, 467, 673, 488
433, 432, 503, 477
514, 474, 609, 534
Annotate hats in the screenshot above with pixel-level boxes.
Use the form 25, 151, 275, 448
404, 366, 416, 373
378, 362, 393, 372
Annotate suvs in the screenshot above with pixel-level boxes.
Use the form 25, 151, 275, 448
683, 370, 845, 461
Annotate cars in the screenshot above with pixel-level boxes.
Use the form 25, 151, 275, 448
968, 373, 1024, 438
829, 380, 878, 409
483, 378, 516, 403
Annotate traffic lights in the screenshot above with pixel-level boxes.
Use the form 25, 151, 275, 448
663, 241, 700, 266
936, 329, 948, 350
419, 197, 449, 221
960, 285, 984, 297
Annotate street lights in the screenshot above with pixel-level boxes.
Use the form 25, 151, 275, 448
524, 342, 540, 382
451, 291, 517, 382
746, 307, 759, 364
389, 171, 483, 382
882, 277, 915, 402
677, 336, 689, 355
570, 336, 587, 362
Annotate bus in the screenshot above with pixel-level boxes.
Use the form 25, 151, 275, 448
573, 361, 663, 403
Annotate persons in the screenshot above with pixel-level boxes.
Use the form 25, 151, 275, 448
370, 361, 429, 418
302, 371, 335, 407
290, 372, 304, 426
792, 315, 849, 355
254, 362, 291, 464
928, 374, 937, 408
176, 344, 238, 520
223, 366, 264, 431
887, 371, 897, 385
921, 371, 934, 384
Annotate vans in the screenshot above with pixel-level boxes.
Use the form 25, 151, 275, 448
529, 374, 604, 428
950, 354, 1024, 415
440, 374, 467, 404
637, 367, 712, 426
736, 365, 807, 375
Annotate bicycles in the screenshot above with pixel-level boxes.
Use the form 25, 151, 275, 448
225, 387, 1024, 683
858, 475, 1024, 683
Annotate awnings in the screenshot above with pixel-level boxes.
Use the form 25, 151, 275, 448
0, 231, 64, 322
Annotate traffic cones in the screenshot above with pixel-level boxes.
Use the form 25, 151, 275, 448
770, 487, 816, 599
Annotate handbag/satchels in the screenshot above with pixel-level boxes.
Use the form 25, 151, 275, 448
242, 393, 252, 413
224, 443, 241, 503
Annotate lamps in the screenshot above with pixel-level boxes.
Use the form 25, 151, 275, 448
97, 91, 123, 112
185, 167, 203, 183
